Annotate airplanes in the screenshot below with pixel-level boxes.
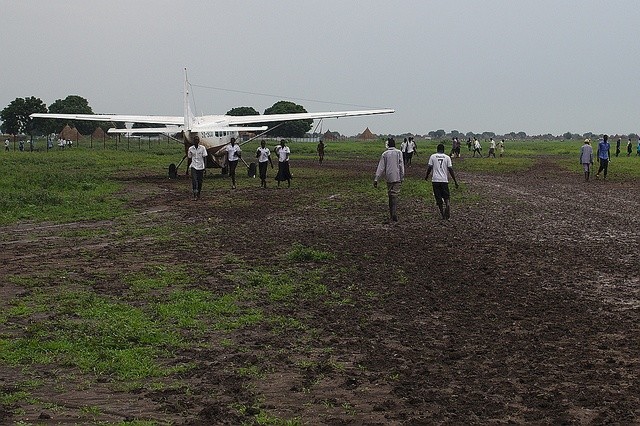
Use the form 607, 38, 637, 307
29, 65, 396, 179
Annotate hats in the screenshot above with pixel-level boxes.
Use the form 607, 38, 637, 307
584, 138, 590, 143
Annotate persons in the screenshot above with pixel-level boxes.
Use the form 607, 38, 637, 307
425, 144, 459, 220
579, 139, 594, 182
466, 137, 484, 158
626, 139, 632, 157
255, 140, 274, 189
48, 137, 73, 150
5, 138, 10, 151
274, 139, 292, 189
317, 139, 327, 166
486, 137, 497, 158
450, 137, 461, 157
400, 137, 419, 167
374, 138, 404, 220
18, 139, 33, 152
226, 138, 242, 189
187, 136, 208, 200
636, 138, 640, 155
496, 139, 505, 157
597, 135, 611, 180
616, 137, 621, 156
